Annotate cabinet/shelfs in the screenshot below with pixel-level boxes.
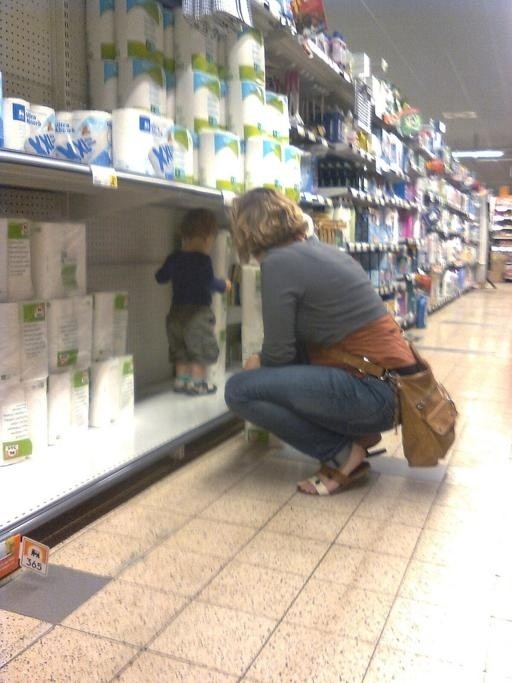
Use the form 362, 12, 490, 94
248, 0, 481, 326
489, 196, 512, 281
0, 149, 245, 577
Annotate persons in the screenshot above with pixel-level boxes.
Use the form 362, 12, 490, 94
226, 189, 417, 498
153, 206, 231, 397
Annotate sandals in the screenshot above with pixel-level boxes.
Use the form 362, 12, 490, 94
171, 376, 190, 392
186, 379, 217, 395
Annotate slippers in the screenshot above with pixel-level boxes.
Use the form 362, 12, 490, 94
297, 463, 371, 494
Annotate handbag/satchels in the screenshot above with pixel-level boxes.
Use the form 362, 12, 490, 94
317, 342, 458, 468
